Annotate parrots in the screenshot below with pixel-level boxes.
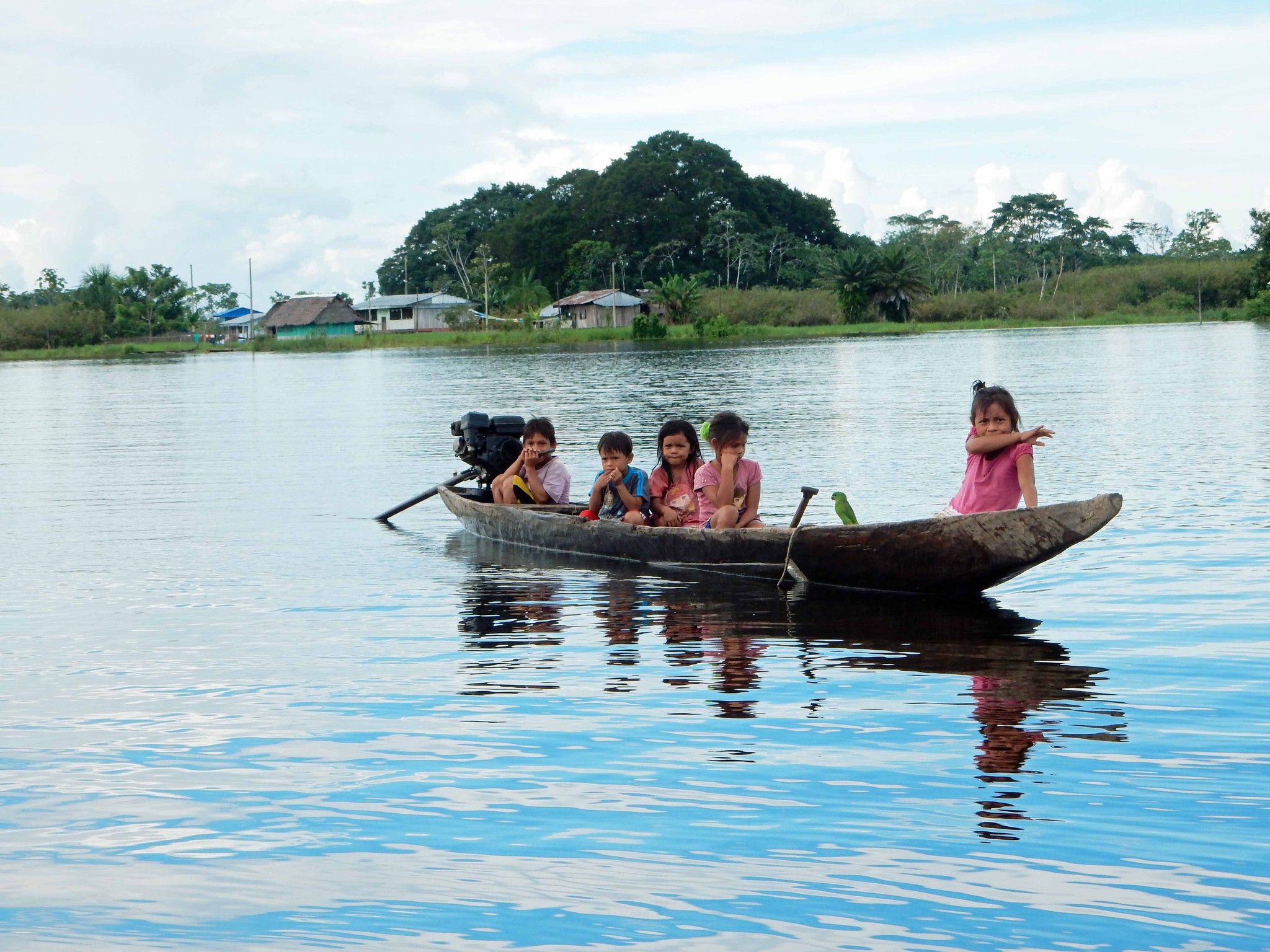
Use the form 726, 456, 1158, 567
831, 492, 859, 525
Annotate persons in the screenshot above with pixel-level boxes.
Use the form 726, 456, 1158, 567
579, 431, 651, 524
933, 380, 1056, 518
491, 417, 572, 505
695, 412, 766, 529
648, 419, 706, 527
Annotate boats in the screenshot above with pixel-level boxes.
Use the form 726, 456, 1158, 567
438, 480, 1123, 597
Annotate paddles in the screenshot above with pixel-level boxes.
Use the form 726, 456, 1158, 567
789, 485, 819, 528
